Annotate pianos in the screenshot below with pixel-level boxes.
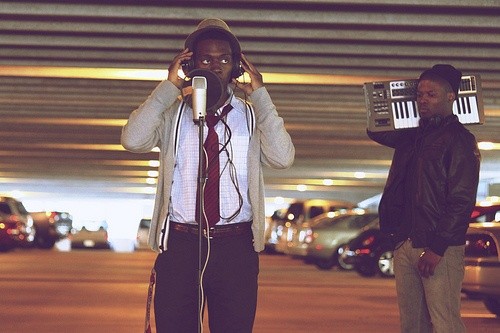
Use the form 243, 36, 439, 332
363, 74, 486, 132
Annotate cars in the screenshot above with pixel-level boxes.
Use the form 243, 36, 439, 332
0, 194, 111, 250
133, 218, 152, 250
265, 198, 499, 279
460, 221, 500, 314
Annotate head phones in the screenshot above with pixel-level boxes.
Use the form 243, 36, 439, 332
181, 58, 245, 81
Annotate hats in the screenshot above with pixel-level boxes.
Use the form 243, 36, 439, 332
431, 64, 463, 100
184, 18, 242, 83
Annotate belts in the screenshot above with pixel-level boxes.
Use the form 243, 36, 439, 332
170, 220, 252, 240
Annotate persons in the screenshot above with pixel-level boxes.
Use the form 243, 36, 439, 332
120, 17, 296, 333
365, 64, 481, 333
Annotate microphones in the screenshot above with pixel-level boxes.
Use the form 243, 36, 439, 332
184, 69, 223, 122
